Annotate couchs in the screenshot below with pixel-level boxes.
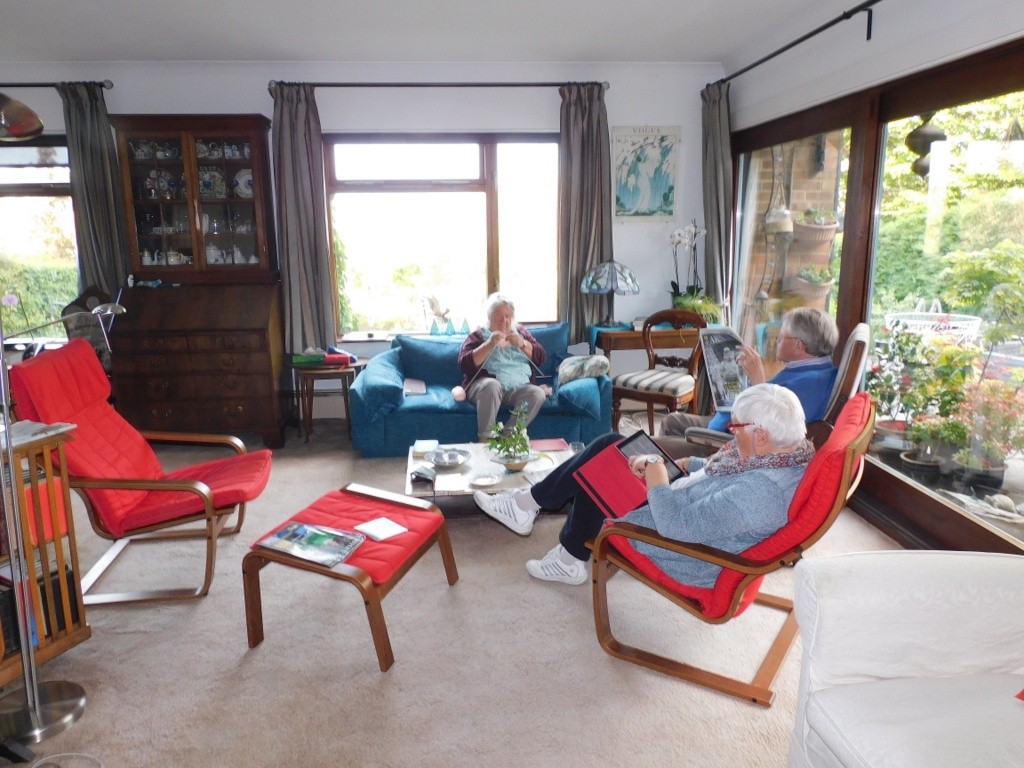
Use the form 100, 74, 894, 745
349, 321, 618, 459
788, 546, 1024, 768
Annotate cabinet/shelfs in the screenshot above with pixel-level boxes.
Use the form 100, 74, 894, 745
104, 111, 287, 446
0, 416, 106, 695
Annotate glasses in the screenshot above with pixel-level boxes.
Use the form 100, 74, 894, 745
728, 420, 762, 432
776, 334, 801, 344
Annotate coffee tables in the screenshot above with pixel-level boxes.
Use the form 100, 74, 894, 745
404, 437, 578, 515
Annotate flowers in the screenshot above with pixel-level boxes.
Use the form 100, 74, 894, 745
480, 400, 532, 458
668, 217, 722, 327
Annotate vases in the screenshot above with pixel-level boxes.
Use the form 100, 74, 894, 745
497, 459, 529, 473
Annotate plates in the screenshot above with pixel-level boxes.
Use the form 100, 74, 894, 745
233, 168, 252, 198
198, 166, 226, 199
143, 167, 176, 193
469, 471, 503, 487
424, 449, 471, 467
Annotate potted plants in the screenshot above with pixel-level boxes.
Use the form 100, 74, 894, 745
874, 319, 1024, 499
792, 263, 837, 298
797, 207, 840, 245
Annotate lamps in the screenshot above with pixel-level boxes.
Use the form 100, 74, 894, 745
579, 258, 642, 329
0, 300, 130, 746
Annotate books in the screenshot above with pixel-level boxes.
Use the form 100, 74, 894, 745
412, 439, 438, 457
404, 377, 426, 396
255, 519, 366, 569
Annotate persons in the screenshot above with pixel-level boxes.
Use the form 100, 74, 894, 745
658, 307, 840, 435
458, 290, 546, 444
472, 382, 815, 589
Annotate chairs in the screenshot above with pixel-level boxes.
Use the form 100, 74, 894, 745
584, 323, 879, 709
9, 335, 275, 606
613, 309, 708, 434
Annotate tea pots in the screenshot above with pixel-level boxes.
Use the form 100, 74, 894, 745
206, 241, 226, 264
138, 246, 191, 266
195, 140, 239, 158
128, 140, 179, 159
222, 244, 248, 264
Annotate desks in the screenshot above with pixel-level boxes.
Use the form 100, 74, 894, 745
591, 317, 700, 371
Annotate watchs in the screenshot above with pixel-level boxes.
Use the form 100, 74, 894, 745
643, 454, 660, 468
520, 339, 528, 349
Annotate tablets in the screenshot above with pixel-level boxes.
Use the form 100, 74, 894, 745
614, 429, 685, 486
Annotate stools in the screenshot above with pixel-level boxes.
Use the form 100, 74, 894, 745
241, 481, 462, 674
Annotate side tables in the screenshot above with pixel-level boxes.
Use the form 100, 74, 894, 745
297, 355, 366, 443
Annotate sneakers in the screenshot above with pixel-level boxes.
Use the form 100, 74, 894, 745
526, 544, 587, 585
474, 489, 539, 535
